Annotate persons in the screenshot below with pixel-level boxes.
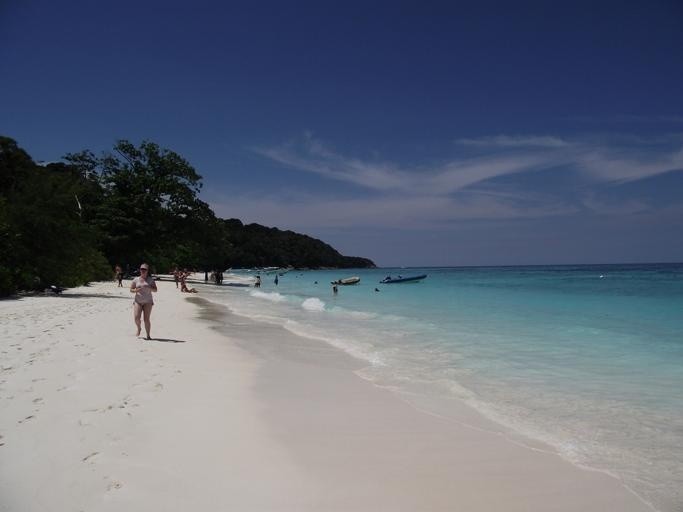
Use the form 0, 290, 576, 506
173, 266, 199, 293
253, 276, 262, 288
332, 281, 338, 295
130, 263, 158, 340
115, 263, 125, 288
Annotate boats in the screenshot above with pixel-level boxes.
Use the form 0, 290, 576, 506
376, 273, 428, 285
330, 276, 363, 285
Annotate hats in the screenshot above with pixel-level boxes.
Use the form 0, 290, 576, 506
138, 262, 150, 271
